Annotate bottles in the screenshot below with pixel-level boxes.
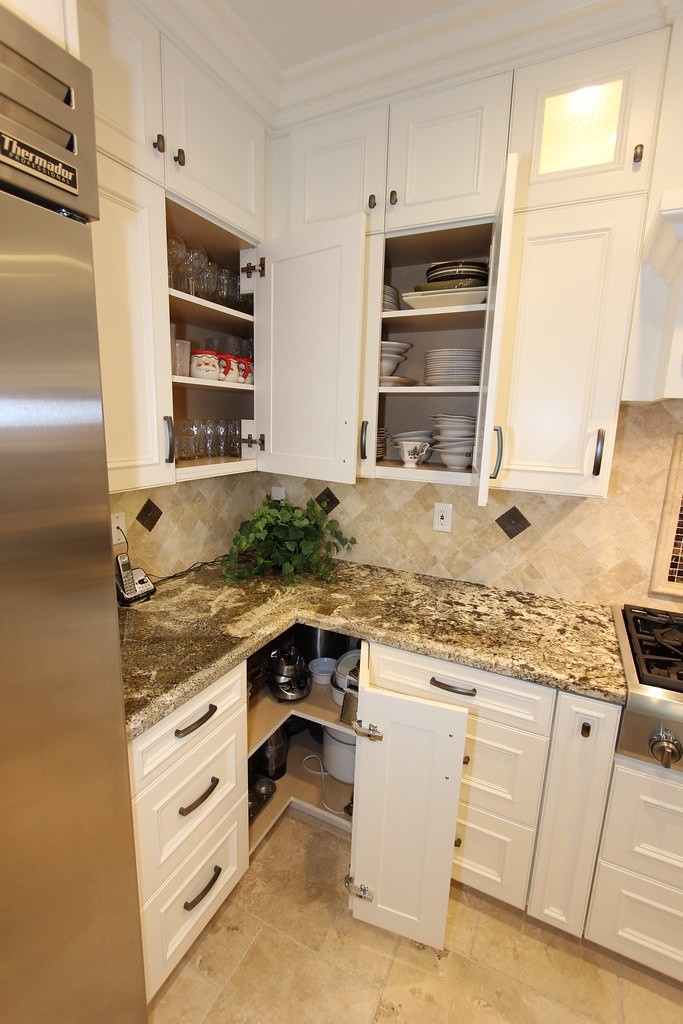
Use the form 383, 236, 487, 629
253, 724, 288, 781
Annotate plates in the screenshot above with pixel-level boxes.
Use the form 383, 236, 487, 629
402, 261, 489, 309
382, 285, 401, 312
380, 377, 414, 386
376, 428, 387, 461
424, 348, 482, 386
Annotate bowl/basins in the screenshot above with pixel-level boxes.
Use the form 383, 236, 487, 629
308, 658, 337, 685
391, 414, 476, 469
380, 342, 412, 376
330, 650, 361, 707
283, 714, 324, 745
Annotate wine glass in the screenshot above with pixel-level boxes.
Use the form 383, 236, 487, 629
168, 235, 207, 297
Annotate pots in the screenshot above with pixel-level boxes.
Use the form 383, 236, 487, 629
322, 725, 356, 785
296, 622, 350, 658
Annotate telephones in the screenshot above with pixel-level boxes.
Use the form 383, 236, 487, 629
116, 553, 156, 600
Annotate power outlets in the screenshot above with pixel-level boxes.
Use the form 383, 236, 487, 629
111, 512, 128, 546
433, 502, 453, 533
271, 487, 286, 504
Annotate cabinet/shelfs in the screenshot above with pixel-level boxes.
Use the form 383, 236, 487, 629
9, 0, 683, 507
125, 623, 683, 1006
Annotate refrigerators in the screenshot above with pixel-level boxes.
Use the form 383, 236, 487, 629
0, 5, 148, 1024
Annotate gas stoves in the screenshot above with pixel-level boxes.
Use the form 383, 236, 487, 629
613, 602, 683, 773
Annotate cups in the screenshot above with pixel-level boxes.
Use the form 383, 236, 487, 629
197, 262, 253, 316
205, 337, 254, 359
174, 419, 241, 464
170, 323, 176, 375
400, 442, 430, 467
190, 350, 254, 385
176, 340, 191, 377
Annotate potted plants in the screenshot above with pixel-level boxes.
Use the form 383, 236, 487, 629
220, 493, 359, 587
